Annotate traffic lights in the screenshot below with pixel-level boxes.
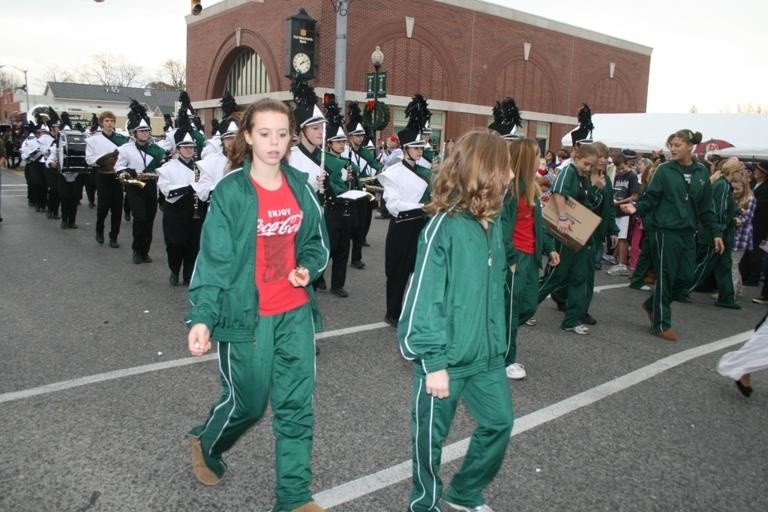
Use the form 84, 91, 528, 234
192, 0, 202, 16
322, 93, 335, 105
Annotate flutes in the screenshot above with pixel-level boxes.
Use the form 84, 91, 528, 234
193, 165, 201, 218
342, 147, 353, 216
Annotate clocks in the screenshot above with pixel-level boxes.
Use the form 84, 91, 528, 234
290, 49, 313, 76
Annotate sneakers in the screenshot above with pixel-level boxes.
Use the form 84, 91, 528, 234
504, 363, 529, 382
27, 202, 179, 287
734, 375, 755, 397
192, 437, 227, 486
314, 259, 367, 298
524, 301, 596, 336
441, 498, 494, 512
608, 251, 741, 340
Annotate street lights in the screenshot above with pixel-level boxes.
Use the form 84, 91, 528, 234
0, 65, 30, 115
371, 45, 383, 159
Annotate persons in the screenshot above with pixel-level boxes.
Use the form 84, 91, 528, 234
536, 129, 767, 339
716, 320, 768, 395
2, 106, 100, 228
287, 104, 456, 355
84, 111, 245, 284
392, 127, 520, 512
179, 95, 332, 512
502, 134, 560, 380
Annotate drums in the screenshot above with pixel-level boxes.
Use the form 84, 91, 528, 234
57, 130, 93, 174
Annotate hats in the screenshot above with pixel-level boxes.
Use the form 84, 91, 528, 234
397, 103, 426, 148
421, 119, 432, 134
125, 103, 241, 150
293, 103, 365, 142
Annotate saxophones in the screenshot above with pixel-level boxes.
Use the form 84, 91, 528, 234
361, 183, 384, 202
119, 172, 160, 189
